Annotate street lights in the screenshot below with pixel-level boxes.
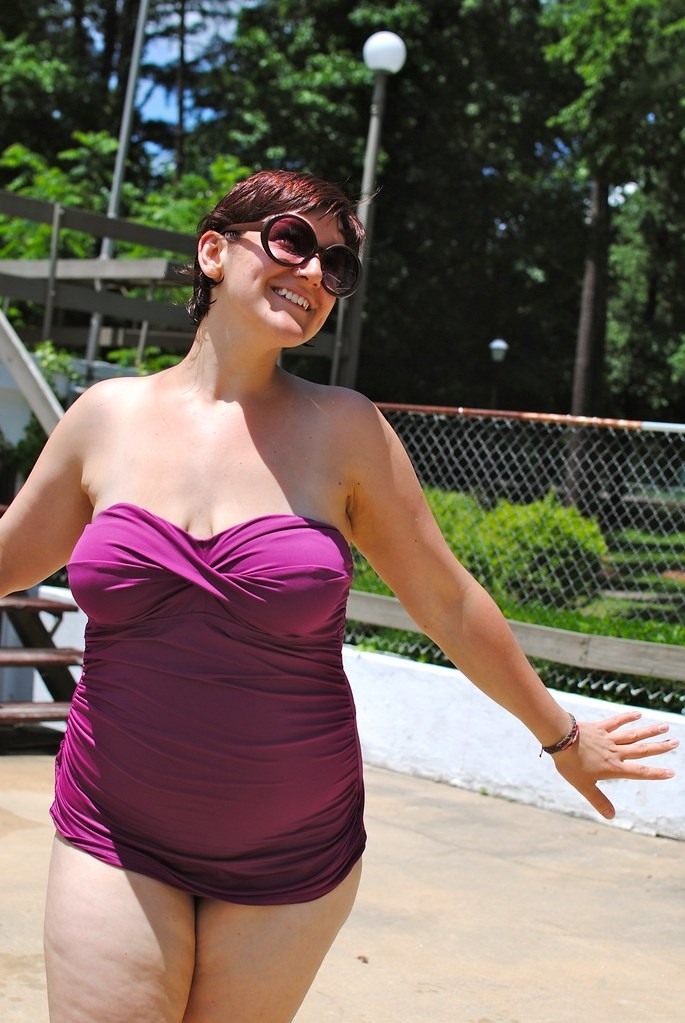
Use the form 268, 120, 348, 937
328, 30, 406, 388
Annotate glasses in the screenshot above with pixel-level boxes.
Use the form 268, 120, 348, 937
219, 212, 364, 300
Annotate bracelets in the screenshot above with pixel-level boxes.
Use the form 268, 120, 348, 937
537, 712, 579, 757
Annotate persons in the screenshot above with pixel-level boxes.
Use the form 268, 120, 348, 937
0, 170, 680, 1023
